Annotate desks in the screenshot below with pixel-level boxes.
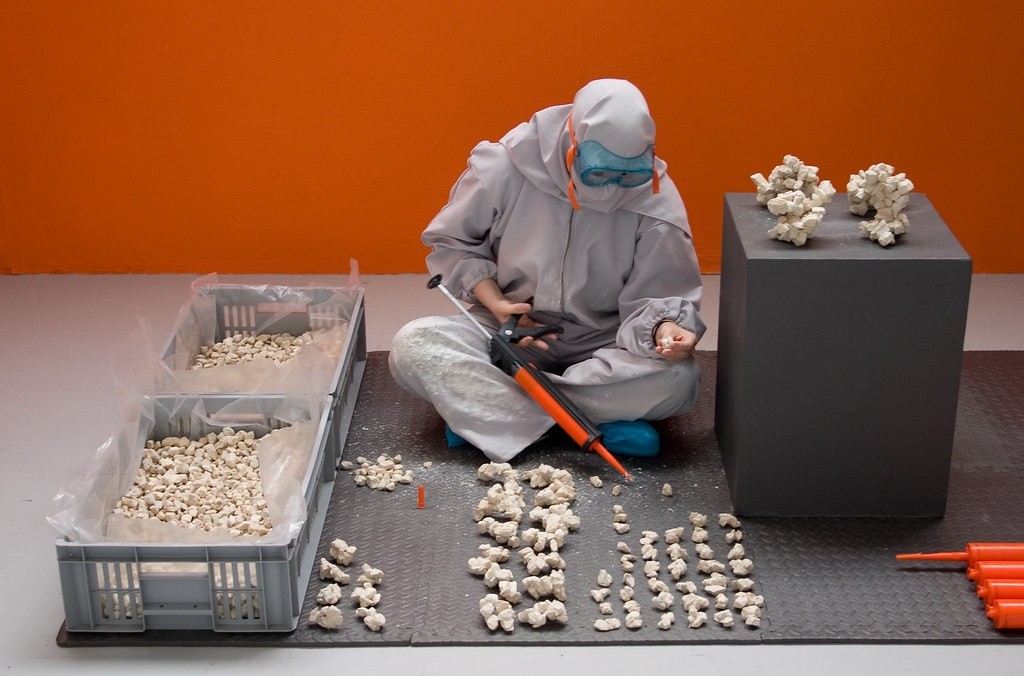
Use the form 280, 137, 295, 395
714, 193, 973, 521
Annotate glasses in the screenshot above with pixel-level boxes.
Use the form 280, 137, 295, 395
573, 140, 656, 188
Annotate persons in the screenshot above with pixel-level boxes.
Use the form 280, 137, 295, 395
388, 79, 707, 462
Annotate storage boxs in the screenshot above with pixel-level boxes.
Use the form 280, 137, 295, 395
52, 394, 342, 631
153, 283, 371, 467
